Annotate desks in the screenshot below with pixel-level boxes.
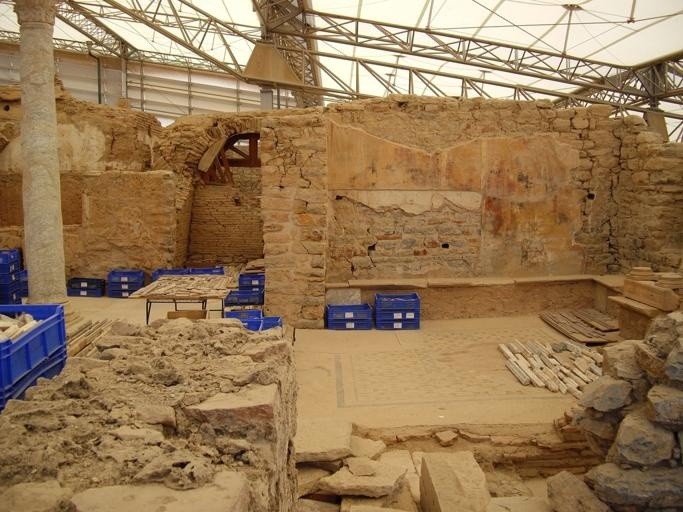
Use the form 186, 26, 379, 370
128, 274, 234, 324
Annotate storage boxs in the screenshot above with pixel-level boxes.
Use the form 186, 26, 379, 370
67, 267, 224, 298
327, 294, 420, 330
0, 250, 67, 412
224, 273, 281, 331
